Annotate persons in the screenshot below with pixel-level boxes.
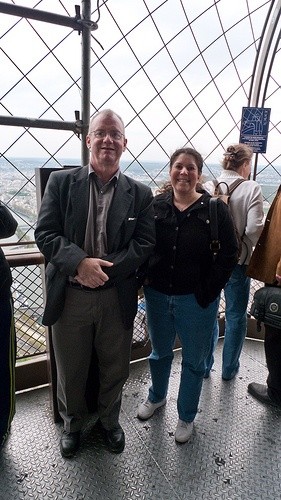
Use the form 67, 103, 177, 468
200, 142, 264, 381
33, 109, 160, 461
137, 146, 240, 444
243, 182, 281, 408
0, 200, 19, 451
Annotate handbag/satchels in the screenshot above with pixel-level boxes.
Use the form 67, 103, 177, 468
249, 278, 281, 333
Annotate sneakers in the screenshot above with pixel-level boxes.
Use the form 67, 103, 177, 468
174, 418, 194, 444
136, 397, 167, 421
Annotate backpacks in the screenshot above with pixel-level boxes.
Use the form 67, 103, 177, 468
211, 178, 246, 262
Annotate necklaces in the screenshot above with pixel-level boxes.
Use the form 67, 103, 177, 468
174, 194, 196, 212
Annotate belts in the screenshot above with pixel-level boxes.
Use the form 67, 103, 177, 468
68, 279, 116, 293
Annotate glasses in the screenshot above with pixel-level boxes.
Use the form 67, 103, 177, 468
89, 128, 125, 141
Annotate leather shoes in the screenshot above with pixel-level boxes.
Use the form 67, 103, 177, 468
247, 382, 281, 409
102, 424, 125, 454
59, 429, 81, 458
